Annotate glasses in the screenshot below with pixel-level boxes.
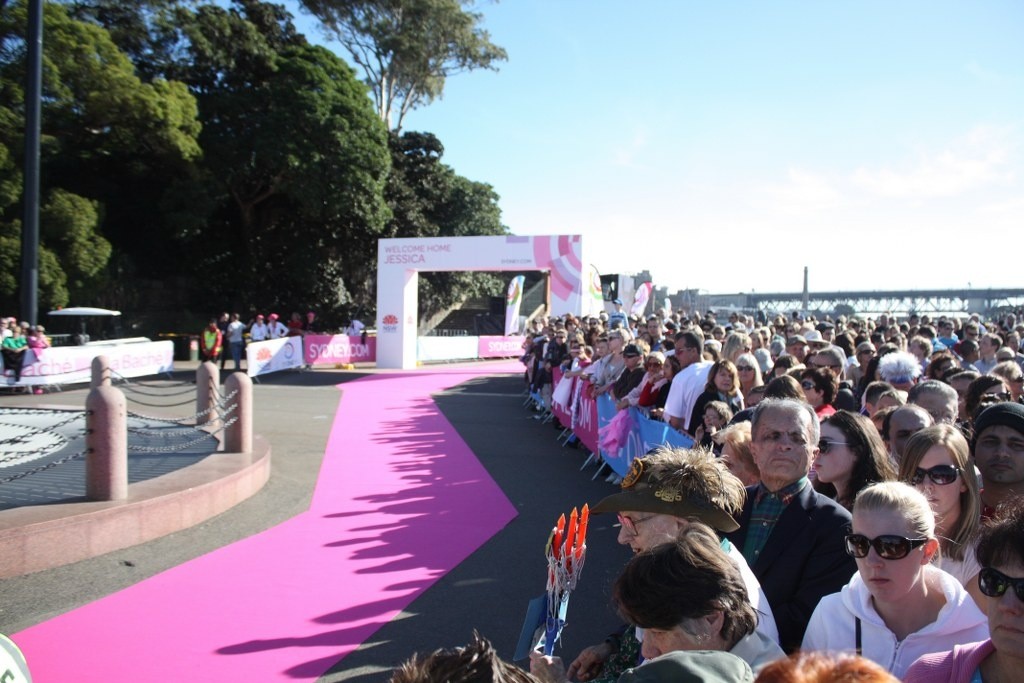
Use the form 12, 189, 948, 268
817, 440, 857, 455
615, 512, 665, 536
646, 361, 660, 367
556, 335, 565, 339
674, 348, 694, 356
803, 381, 819, 390
702, 329, 710, 332
944, 328, 954, 331
608, 337, 620, 341
1009, 375, 1024, 383
742, 346, 754, 353
980, 391, 1012, 402
910, 466, 959, 485
977, 567, 1024, 601
844, 532, 927, 560
857, 350, 877, 355
966, 333, 979, 337
622, 354, 640, 358
589, 322, 597, 326
737, 365, 755, 372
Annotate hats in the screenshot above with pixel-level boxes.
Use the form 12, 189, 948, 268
803, 330, 831, 346
620, 344, 643, 356
588, 447, 741, 533
970, 403, 1024, 454
754, 348, 774, 372
787, 336, 807, 347
613, 298, 622, 306
616, 650, 752, 683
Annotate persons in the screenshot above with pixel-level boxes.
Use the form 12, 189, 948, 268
521, 297, 1022, 683
198, 305, 368, 373
0, 315, 50, 397
